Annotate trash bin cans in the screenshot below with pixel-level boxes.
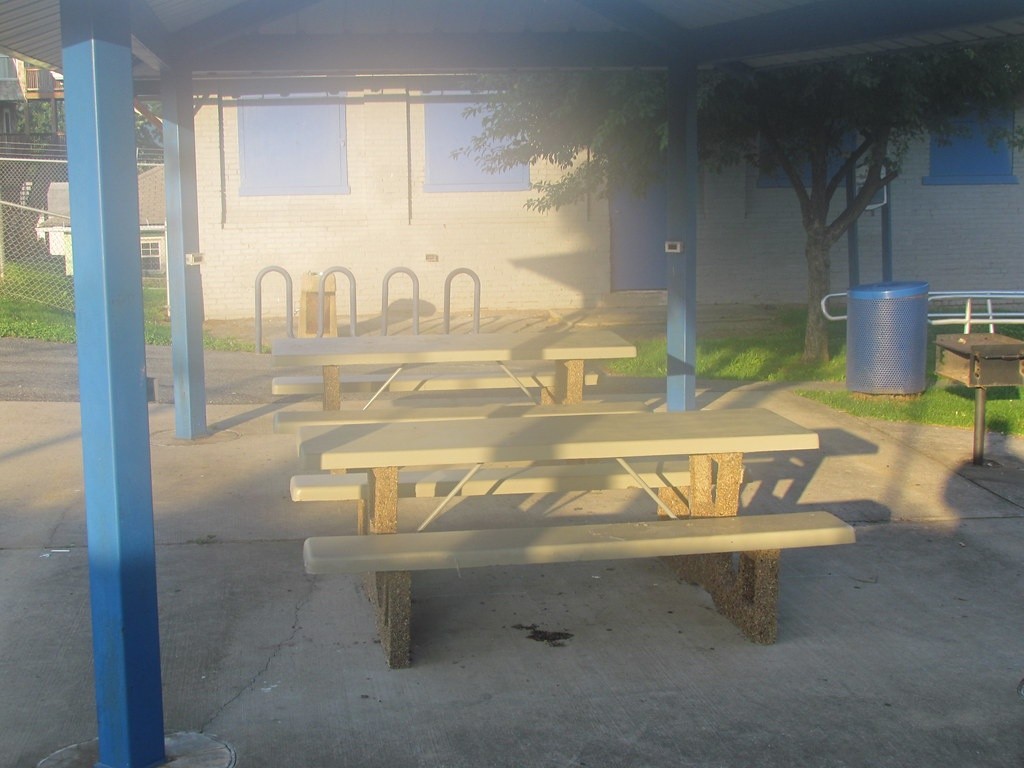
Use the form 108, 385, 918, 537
298, 272, 338, 337
843, 280, 931, 395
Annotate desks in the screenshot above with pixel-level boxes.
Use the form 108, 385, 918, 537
270, 329, 637, 474
296, 405, 821, 670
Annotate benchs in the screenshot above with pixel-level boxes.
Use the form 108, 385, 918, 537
302, 510, 858, 577
270, 367, 599, 396
273, 401, 653, 435
289, 459, 745, 501
